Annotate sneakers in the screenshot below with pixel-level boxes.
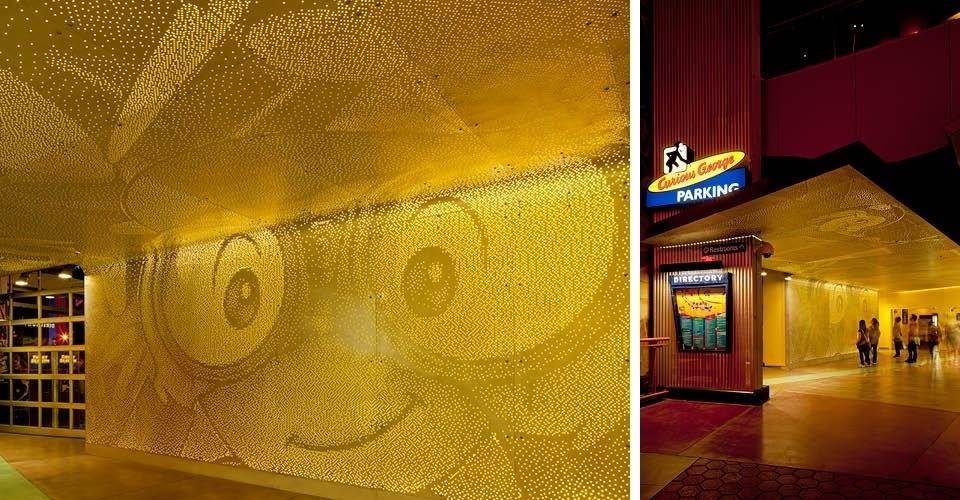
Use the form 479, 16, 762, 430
904, 358, 916, 363
865, 362, 871, 367
857, 363, 865, 368
893, 354, 900, 358
870, 360, 879, 364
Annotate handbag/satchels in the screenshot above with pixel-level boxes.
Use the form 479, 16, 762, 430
899, 340, 904, 350
867, 341, 872, 351
913, 337, 920, 346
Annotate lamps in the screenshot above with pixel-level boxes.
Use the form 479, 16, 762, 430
58, 265, 75, 279
15, 271, 33, 287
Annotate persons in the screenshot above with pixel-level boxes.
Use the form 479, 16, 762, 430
893, 316, 903, 359
855, 319, 873, 369
903, 313, 919, 363
926, 319, 941, 358
868, 317, 881, 364
